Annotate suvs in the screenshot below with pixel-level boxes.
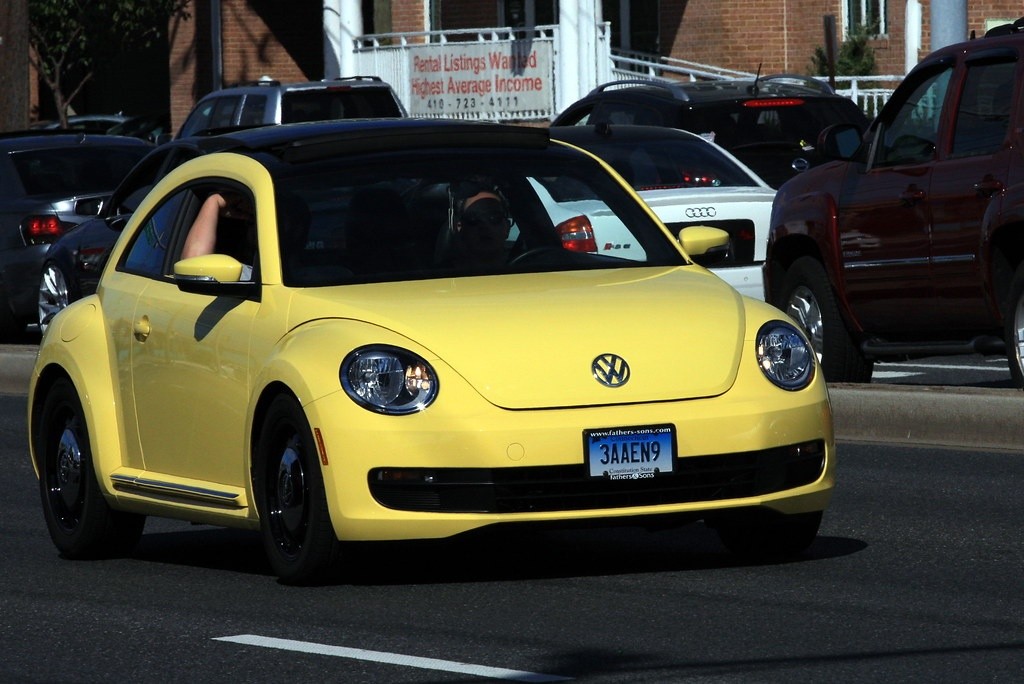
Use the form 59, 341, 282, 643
168, 71, 405, 151
545, 69, 875, 195
760, 14, 1024, 393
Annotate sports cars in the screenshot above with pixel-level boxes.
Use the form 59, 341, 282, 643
27, 116, 839, 594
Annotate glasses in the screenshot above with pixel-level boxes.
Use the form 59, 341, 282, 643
465, 211, 506, 227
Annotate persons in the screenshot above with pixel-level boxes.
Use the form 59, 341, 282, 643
418, 191, 588, 280
180, 191, 349, 282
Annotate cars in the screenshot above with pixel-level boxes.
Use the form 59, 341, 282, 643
0, 113, 170, 339
484, 118, 783, 310
36, 132, 236, 341
